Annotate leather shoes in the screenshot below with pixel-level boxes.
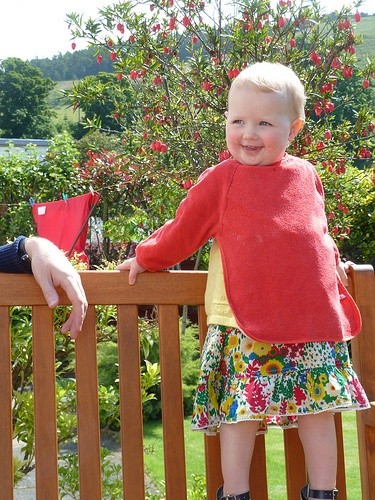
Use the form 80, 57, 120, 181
301, 483, 338, 500
216, 485, 250, 500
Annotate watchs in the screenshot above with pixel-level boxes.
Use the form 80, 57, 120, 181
18, 238, 31, 268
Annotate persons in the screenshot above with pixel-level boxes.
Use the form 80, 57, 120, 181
0, 235, 88, 340
113, 61, 370, 500
